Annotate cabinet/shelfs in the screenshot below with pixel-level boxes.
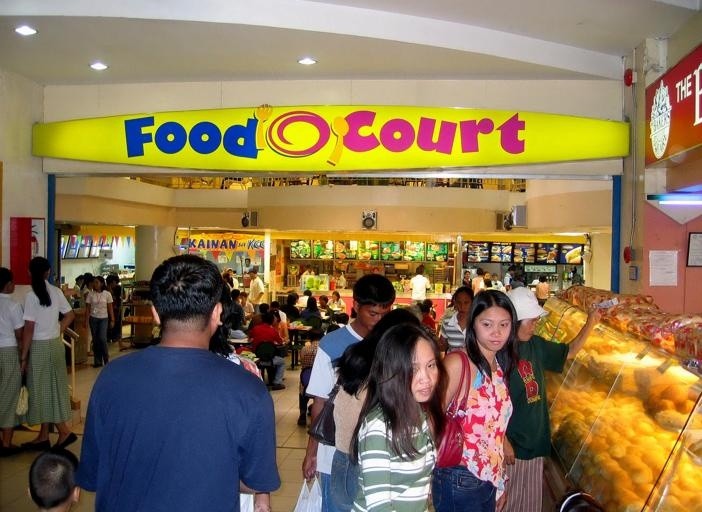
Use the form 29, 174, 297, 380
533, 295, 702, 512
119, 279, 163, 353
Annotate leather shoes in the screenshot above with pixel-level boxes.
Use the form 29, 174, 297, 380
22, 440, 51, 452
0, 439, 23, 457
51, 431, 78, 450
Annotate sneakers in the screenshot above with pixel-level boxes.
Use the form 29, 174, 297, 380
93, 358, 109, 368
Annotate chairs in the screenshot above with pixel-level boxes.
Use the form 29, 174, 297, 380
227, 302, 348, 386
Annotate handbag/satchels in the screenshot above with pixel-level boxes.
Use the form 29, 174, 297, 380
307, 401, 335, 446
433, 410, 463, 468
15, 386, 29, 416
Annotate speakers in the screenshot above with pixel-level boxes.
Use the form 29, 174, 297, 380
241, 208, 259, 228
495, 209, 512, 231
361, 208, 377, 233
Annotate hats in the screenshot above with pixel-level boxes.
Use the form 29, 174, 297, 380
505, 286, 549, 322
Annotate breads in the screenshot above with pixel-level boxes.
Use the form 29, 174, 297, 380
534, 284, 702, 512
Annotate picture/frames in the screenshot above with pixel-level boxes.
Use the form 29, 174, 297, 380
684, 230, 702, 268
